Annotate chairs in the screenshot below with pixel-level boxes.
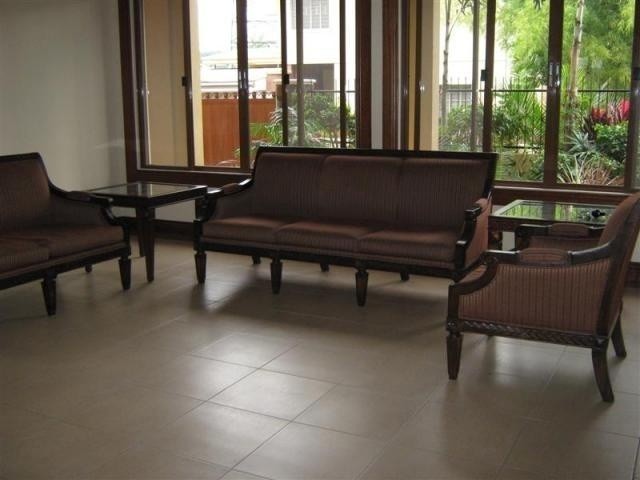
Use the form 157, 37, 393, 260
445, 191, 640, 402
0, 152, 131, 316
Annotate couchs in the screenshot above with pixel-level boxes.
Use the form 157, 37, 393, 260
192, 147, 501, 305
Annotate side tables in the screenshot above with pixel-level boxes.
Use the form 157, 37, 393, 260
83, 181, 208, 282
488, 197, 618, 250
